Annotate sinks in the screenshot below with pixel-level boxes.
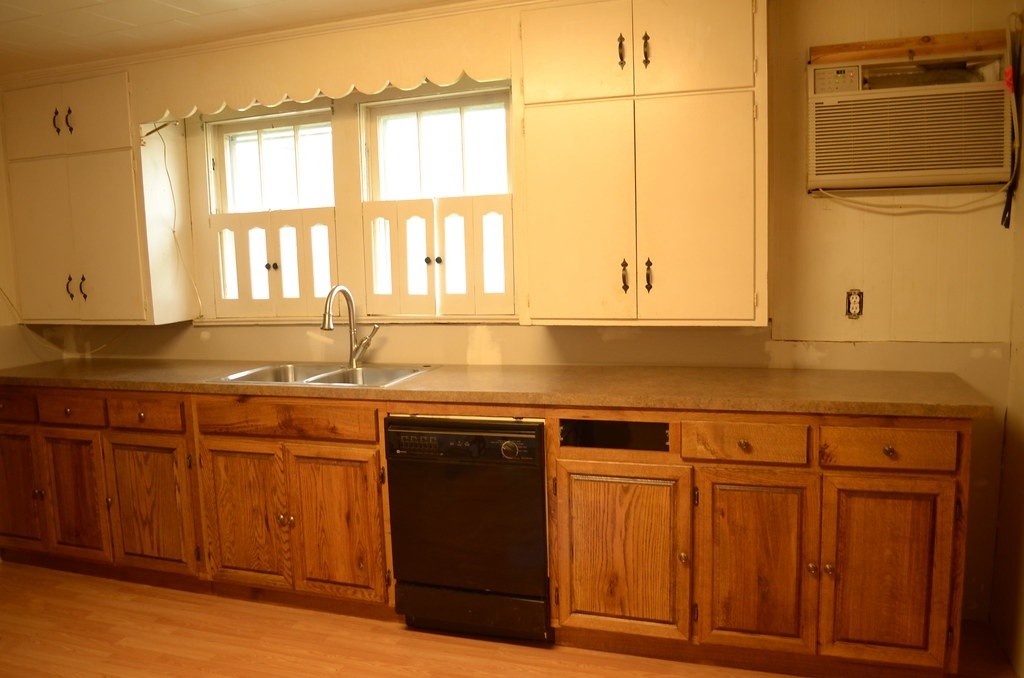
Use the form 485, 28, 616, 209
303, 360, 443, 388
203, 362, 345, 384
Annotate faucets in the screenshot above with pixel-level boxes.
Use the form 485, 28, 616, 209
320, 285, 358, 366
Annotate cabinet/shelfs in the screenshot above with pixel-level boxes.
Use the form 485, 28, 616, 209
7, 147, 200, 326
513, 89, 768, 327
1, 70, 183, 161
553, 362, 697, 641
0, 356, 117, 564
103, 357, 200, 579
697, 367, 969, 677
194, 358, 391, 604
517, 0, 759, 105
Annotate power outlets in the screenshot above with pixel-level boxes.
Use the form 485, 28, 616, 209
845, 288, 864, 320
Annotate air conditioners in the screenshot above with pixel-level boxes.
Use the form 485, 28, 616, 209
803, 28, 1017, 200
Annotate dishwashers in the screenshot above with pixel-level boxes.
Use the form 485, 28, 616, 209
383, 412, 549, 643
